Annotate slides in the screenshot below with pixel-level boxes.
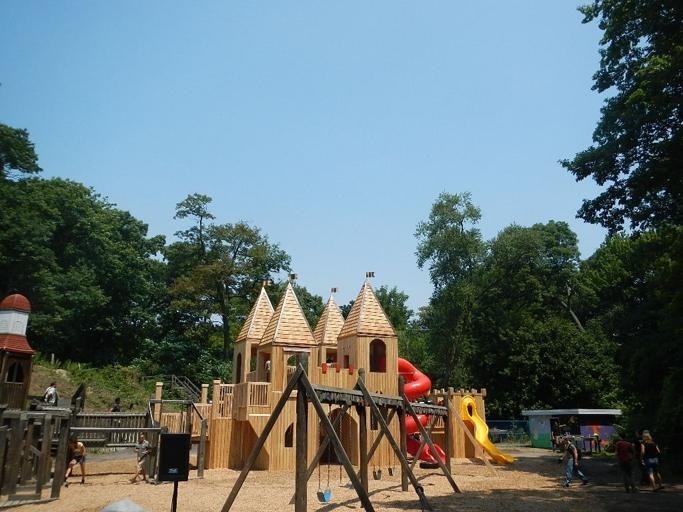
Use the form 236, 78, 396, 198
398, 355, 447, 465
459, 395, 516, 463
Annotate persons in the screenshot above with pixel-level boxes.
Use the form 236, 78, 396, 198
264, 359, 271, 382
42, 381, 58, 406
65, 436, 86, 487
557, 439, 589, 487
128, 433, 154, 484
613, 428, 663, 492
110, 398, 122, 413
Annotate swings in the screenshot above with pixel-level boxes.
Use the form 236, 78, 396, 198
316, 401, 332, 503
371, 410, 384, 480
388, 407, 396, 477
339, 404, 352, 489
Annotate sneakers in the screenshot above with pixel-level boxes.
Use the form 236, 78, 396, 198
564, 479, 666, 491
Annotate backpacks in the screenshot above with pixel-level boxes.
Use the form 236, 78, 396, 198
567, 442, 581, 461
